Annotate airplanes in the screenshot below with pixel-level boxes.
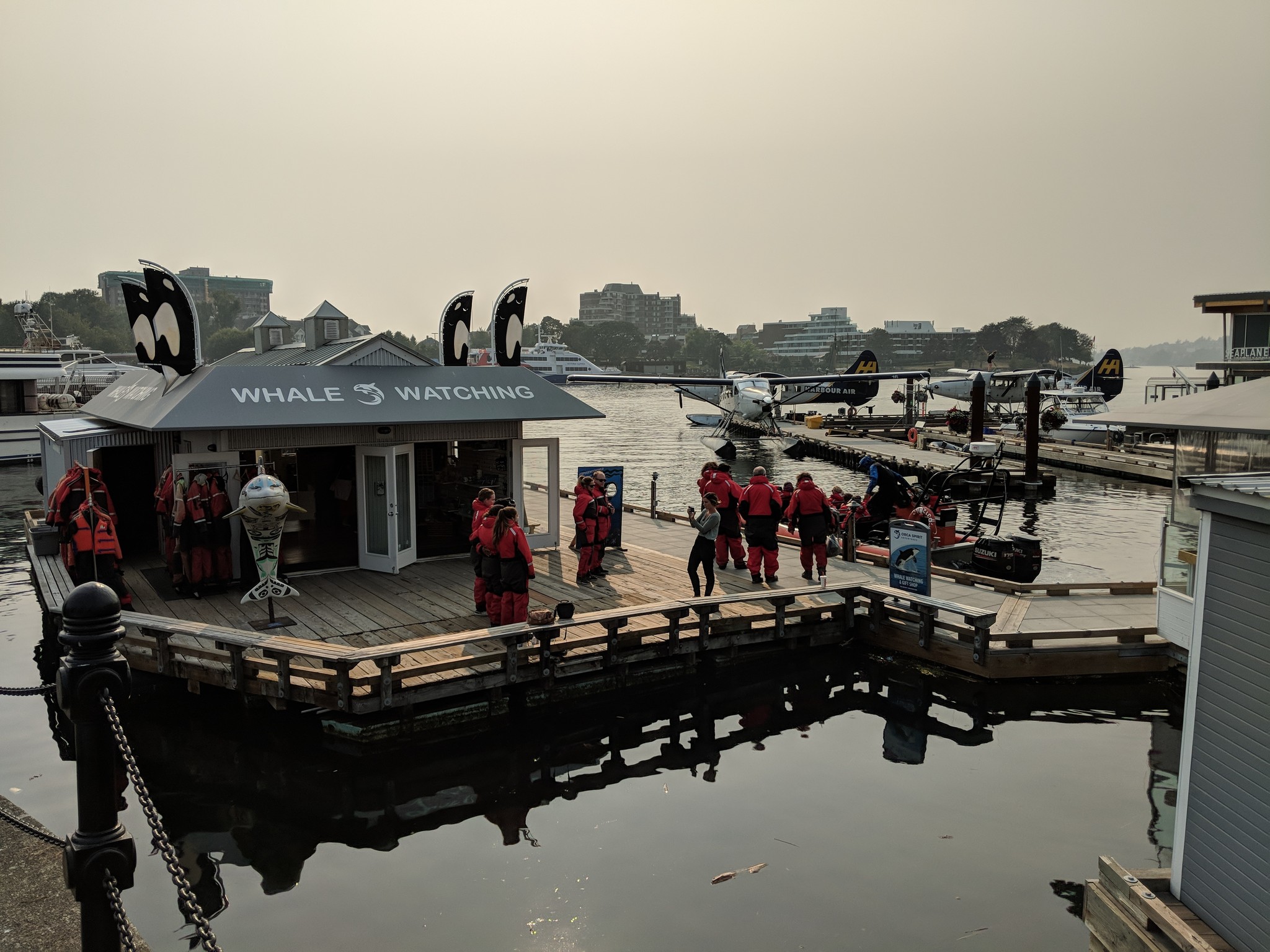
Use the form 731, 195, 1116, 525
922, 349, 1124, 406
674, 343, 879, 461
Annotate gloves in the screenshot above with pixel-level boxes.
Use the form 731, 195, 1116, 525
910, 487, 917, 494
788, 521, 793, 529
829, 525, 837, 534
864, 493, 870, 500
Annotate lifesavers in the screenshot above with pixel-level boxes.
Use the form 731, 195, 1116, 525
908, 506, 937, 535
908, 428, 918, 443
848, 407, 857, 416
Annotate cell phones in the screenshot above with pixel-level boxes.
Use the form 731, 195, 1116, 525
689, 507, 694, 513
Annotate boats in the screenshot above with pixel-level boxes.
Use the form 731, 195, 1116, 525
467, 325, 623, 384
0, 290, 151, 465
998, 387, 1126, 444
735, 442, 1042, 583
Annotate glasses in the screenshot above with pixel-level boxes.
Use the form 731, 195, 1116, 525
831, 490, 834, 492
595, 478, 607, 482
729, 470, 732, 473
589, 483, 597, 488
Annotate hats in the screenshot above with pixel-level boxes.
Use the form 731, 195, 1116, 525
494, 498, 511, 507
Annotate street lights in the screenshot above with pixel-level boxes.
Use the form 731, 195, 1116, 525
699, 352, 701, 374
653, 350, 657, 374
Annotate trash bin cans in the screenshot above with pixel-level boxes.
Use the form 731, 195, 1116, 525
805, 414, 823, 429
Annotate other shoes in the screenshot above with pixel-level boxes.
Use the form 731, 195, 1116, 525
590, 570, 606, 577
597, 567, 609, 574
529, 634, 533, 640
765, 575, 778, 583
802, 571, 812, 580
752, 577, 763, 584
734, 563, 748, 569
818, 574, 826, 581
584, 572, 598, 581
474, 609, 488, 616
719, 562, 728, 570
576, 576, 590, 584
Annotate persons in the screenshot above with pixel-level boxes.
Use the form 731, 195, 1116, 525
784, 473, 834, 582
685, 493, 721, 598
568, 473, 615, 586
987, 351, 997, 371
470, 487, 536, 627
775, 457, 917, 536
738, 466, 783, 585
699, 462, 748, 571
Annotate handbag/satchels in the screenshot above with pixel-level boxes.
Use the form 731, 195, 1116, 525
553, 600, 575, 619
526, 608, 554, 625
826, 533, 841, 558
